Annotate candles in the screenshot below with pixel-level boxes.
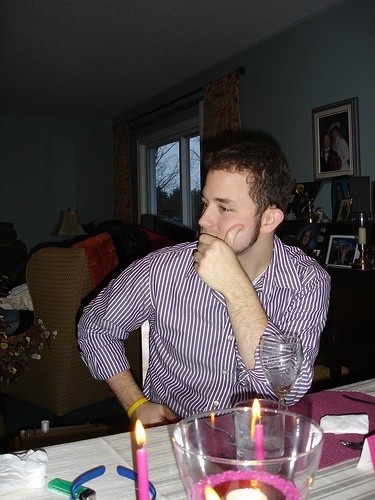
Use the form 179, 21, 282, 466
41, 420, 49, 434
358, 227, 366, 244
254, 398, 264, 470
135, 419, 149, 500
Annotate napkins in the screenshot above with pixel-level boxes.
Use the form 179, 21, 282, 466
320, 414, 369, 435
0, 449, 48, 495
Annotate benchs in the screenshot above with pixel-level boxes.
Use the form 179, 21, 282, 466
0, 379, 375, 500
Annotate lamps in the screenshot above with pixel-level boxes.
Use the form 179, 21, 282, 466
51, 208, 89, 240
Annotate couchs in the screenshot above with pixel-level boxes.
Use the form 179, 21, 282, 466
0, 220, 141, 417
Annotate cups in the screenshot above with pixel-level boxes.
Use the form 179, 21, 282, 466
233, 399, 288, 476
170, 404, 324, 500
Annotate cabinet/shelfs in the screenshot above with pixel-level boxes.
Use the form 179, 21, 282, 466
275, 223, 375, 386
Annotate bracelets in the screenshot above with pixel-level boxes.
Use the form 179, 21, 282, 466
127, 399, 148, 418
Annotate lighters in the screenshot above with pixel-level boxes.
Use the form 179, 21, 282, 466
48, 479, 96, 500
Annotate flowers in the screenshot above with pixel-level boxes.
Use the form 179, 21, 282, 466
0, 315, 57, 391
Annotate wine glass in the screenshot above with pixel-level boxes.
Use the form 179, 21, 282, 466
259, 332, 304, 442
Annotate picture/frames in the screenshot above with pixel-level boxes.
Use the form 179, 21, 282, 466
331, 175, 351, 221
289, 182, 321, 209
337, 199, 353, 221
325, 234, 359, 269
312, 97, 360, 181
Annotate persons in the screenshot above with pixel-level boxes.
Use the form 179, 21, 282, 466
278, 182, 351, 266
319, 121, 350, 172
78, 143, 332, 430
